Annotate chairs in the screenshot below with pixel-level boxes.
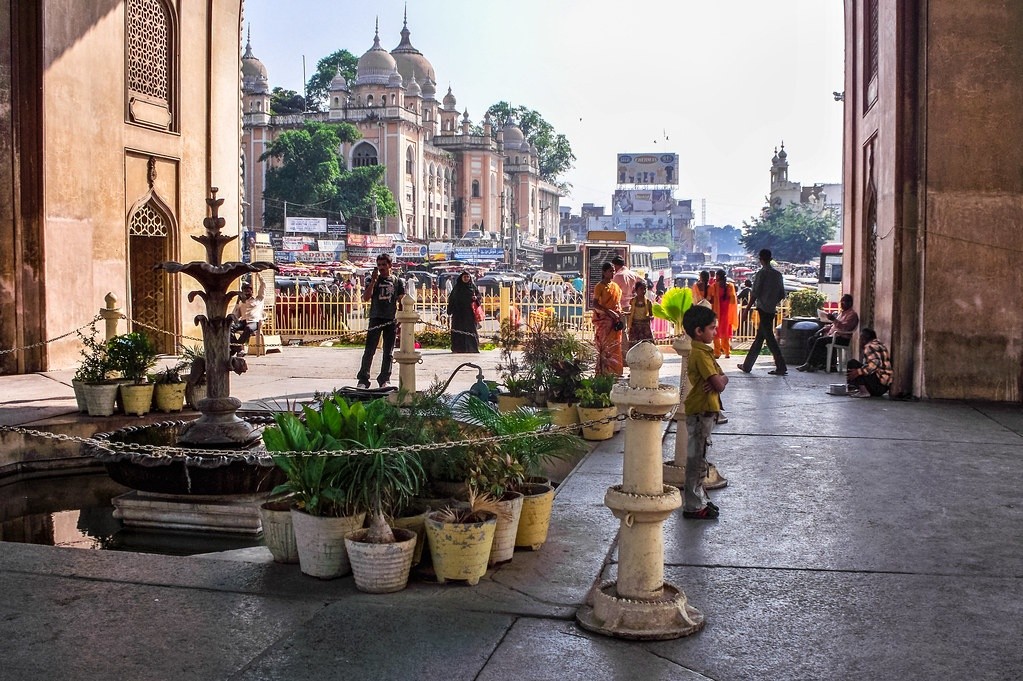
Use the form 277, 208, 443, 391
816, 323, 859, 373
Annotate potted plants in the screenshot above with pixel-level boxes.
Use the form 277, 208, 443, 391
496, 319, 623, 440
69, 319, 206, 417
256, 380, 589, 590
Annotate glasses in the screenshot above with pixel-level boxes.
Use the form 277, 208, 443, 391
377, 263, 388, 267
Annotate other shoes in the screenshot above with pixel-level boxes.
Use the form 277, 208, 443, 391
847, 389, 870, 398
381, 381, 393, 388
237, 350, 246, 358
768, 370, 787, 375
357, 384, 366, 389
737, 364, 749, 373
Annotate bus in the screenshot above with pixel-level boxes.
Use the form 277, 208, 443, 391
817, 243, 844, 315
543, 244, 652, 295
720, 252, 746, 265
686, 252, 710, 264
648, 245, 674, 294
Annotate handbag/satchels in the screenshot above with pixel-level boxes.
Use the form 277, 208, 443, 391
471, 292, 486, 322
614, 320, 623, 331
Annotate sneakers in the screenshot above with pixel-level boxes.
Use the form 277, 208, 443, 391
683, 502, 719, 519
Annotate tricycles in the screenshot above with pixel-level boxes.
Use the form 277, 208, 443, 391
277, 262, 527, 317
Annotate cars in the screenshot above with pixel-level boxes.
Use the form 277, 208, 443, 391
675, 264, 815, 323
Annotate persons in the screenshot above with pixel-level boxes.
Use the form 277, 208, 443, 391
795, 293, 861, 373
843, 327, 895, 401
680, 302, 731, 522
626, 280, 657, 353
355, 253, 406, 393
611, 255, 641, 347
273, 247, 820, 315
736, 248, 790, 377
227, 270, 267, 357
446, 271, 485, 354
590, 261, 627, 385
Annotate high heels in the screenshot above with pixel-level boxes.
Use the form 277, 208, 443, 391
807, 366, 819, 373
796, 362, 810, 372
